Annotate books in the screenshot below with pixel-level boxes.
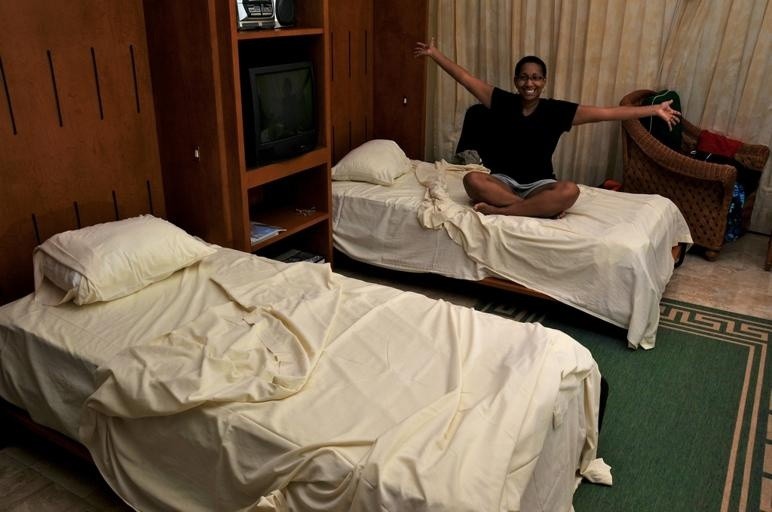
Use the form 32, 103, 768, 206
250, 219, 323, 273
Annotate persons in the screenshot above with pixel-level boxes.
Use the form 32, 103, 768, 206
412, 37, 682, 216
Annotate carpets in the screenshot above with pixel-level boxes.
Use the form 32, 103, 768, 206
463, 295, 772, 512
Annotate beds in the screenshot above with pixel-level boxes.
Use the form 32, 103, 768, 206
331, 155, 695, 353
1, 246, 608, 512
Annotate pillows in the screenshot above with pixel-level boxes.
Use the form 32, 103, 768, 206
25, 210, 219, 305
646, 92, 683, 154
330, 138, 411, 186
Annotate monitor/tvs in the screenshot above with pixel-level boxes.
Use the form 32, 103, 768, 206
246, 61, 320, 168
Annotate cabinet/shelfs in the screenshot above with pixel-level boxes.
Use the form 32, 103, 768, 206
218, 1, 332, 269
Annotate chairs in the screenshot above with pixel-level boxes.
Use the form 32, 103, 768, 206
618, 88, 770, 262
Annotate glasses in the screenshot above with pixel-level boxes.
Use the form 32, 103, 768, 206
517, 74, 543, 82
